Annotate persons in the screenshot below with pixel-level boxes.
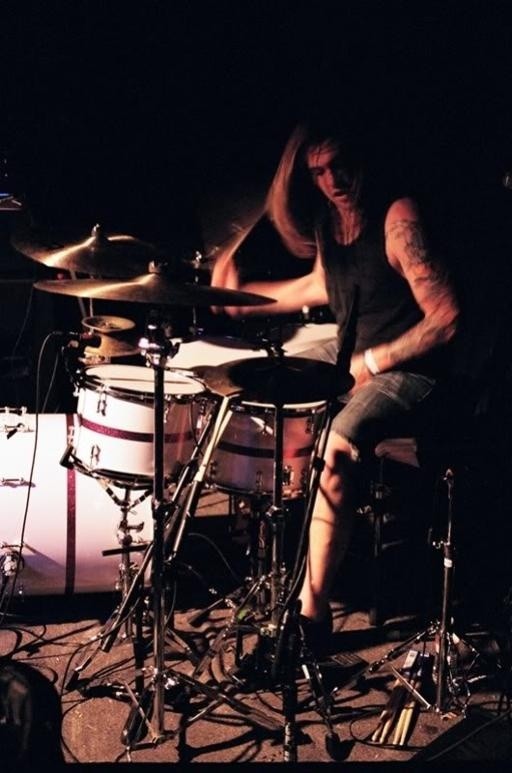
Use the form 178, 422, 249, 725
210, 109, 490, 695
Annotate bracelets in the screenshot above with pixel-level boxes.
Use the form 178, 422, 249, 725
366, 348, 382, 377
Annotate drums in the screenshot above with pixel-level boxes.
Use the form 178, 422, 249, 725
201, 395, 329, 503
145, 333, 285, 376
64, 365, 205, 489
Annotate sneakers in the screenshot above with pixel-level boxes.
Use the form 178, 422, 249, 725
228, 608, 332, 693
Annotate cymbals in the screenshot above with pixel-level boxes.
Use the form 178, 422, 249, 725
9, 221, 155, 279
207, 354, 356, 404
31, 263, 277, 309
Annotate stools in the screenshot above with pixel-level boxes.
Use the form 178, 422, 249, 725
331, 402, 456, 622
